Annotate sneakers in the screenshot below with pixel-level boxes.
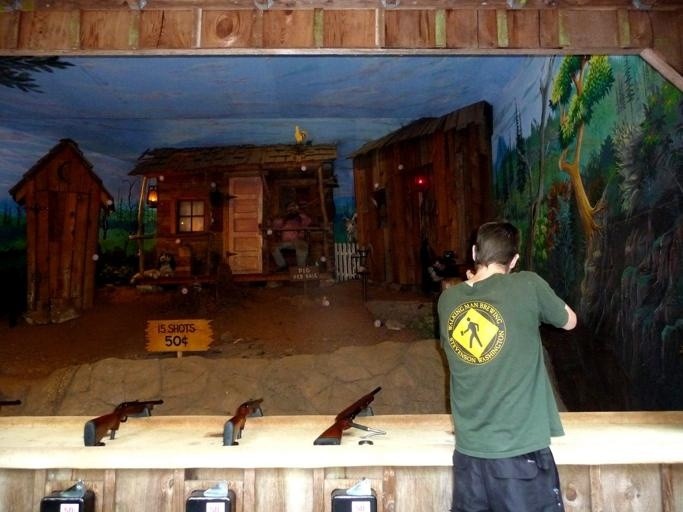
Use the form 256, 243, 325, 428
276, 265, 289, 273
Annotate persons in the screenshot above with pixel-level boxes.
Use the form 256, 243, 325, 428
268, 200, 313, 273
436, 220, 578, 512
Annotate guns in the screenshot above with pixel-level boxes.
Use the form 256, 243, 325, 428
83, 399, 165, 446
224, 398, 264, 447
314, 386, 386, 446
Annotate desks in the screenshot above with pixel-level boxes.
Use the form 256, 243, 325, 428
263, 226, 325, 268
